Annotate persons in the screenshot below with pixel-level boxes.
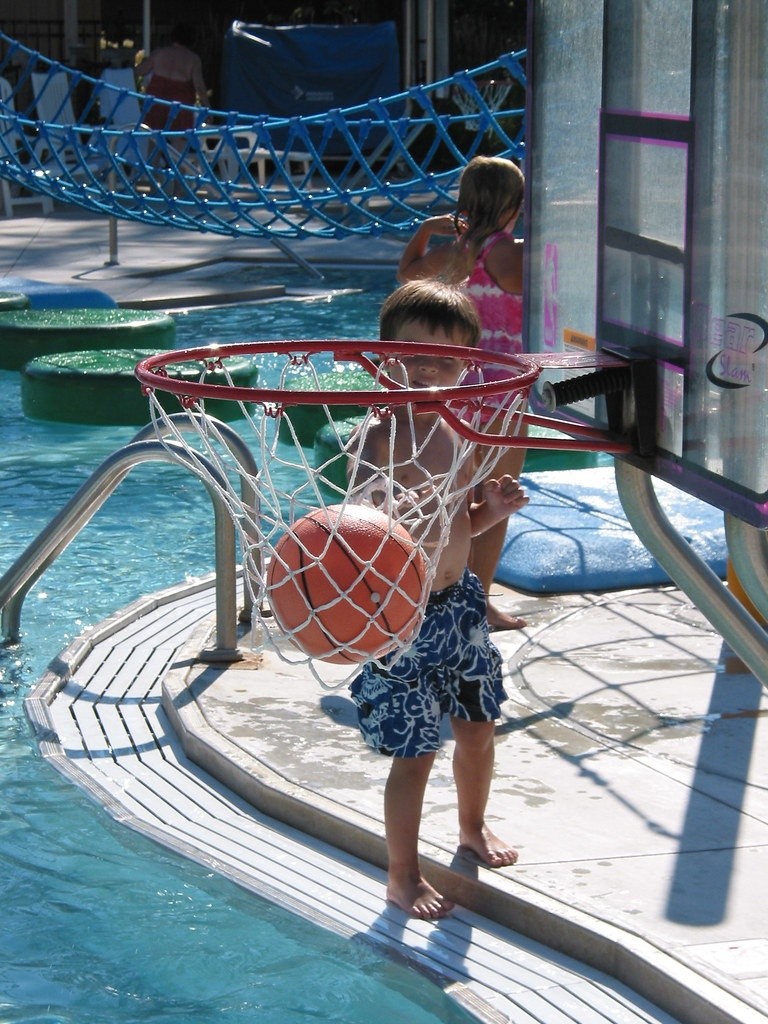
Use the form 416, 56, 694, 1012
345, 276, 530, 920
394, 155, 527, 629
136, 23, 214, 199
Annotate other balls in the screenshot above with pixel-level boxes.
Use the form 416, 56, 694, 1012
267, 501, 430, 666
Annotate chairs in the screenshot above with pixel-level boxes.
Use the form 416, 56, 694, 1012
32, 72, 127, 191
98, 69, 169, 184
194, 123, 314, 190
0, 77, 65, 218
